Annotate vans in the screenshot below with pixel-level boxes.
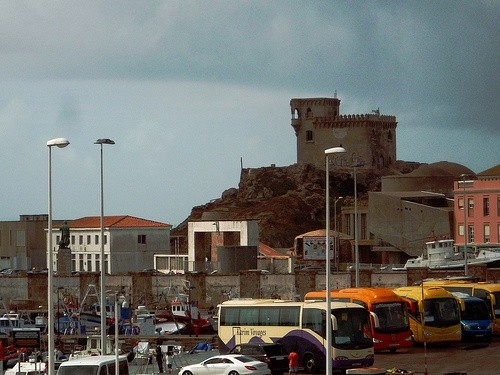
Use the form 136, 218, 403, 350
55, 354, 130, 375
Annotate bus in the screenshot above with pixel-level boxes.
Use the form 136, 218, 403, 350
391, 280, 500, 347
303, 286, 416, 355
216, 297, 376, 374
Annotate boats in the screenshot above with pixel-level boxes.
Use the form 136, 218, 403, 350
0, 294, 209, 336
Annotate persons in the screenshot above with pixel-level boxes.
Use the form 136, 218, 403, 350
126, 349, 135, 362
155, 345, 173, 374
287, 349, 299, 375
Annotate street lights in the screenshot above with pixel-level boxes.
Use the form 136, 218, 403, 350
350, 150, 366, 288
93, 137, 116, 357
46, 137, 72, 375
333, 195, 344, 273
459, 173, 472, 276
324, 146, 348, 375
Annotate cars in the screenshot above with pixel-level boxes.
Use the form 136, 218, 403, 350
177, 353, 273, 375
